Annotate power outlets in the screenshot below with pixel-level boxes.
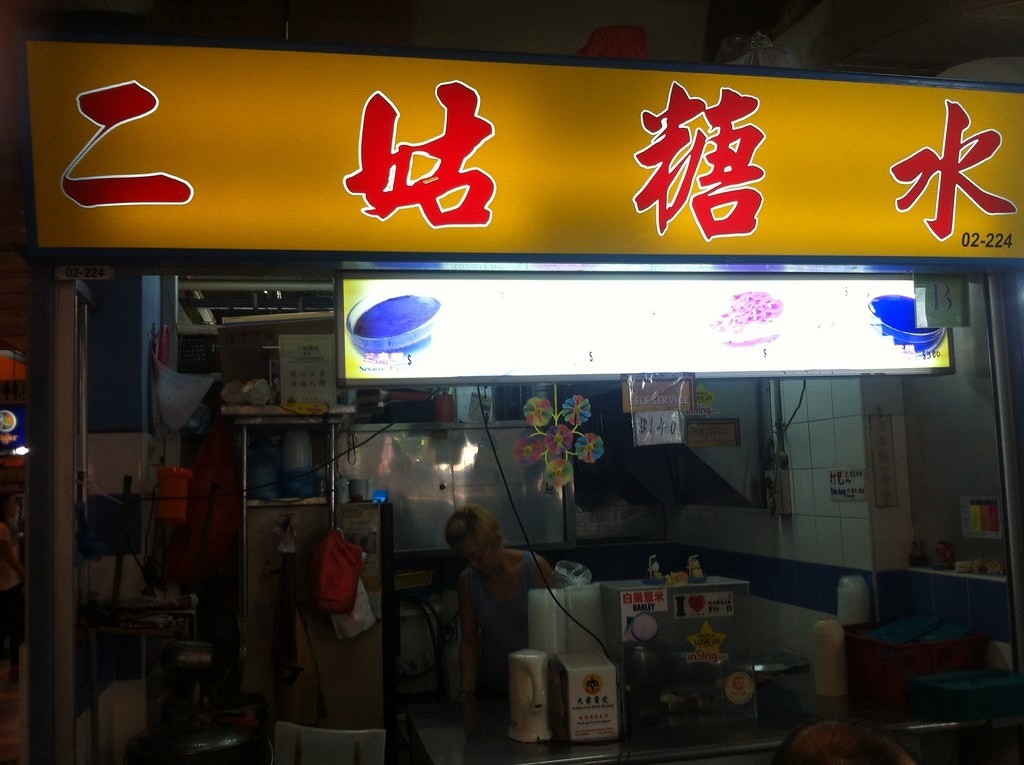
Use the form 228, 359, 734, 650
440, 616, 458, 642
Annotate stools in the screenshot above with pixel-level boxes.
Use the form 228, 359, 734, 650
87, 604, 187, 765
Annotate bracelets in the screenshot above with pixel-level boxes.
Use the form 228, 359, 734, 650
459, 689, 474, 693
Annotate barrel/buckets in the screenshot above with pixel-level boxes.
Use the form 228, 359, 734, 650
156, 467, 192, 524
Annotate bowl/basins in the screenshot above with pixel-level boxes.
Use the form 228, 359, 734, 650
811, 576, 869, 695
527, 583, 605, 659
655, 694, 688, 714
284, 429, 312, 468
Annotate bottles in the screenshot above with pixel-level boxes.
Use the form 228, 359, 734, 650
312, 463, 326, 497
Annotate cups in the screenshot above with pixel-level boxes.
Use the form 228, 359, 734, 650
434, 395, 453, 422
697, 695, 712, 713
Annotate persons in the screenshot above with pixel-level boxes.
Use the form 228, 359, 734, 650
444, 504, 555, 704
0, 492, 25, 679
771, 715, 920, 765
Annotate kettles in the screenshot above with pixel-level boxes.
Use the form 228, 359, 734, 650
508, 649, 554, 742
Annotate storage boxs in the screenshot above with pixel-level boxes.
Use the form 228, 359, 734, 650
178, 334, 220, 373
384, 400, 435, 423
843, 621, 992, 705
216, 317, 335, 370
